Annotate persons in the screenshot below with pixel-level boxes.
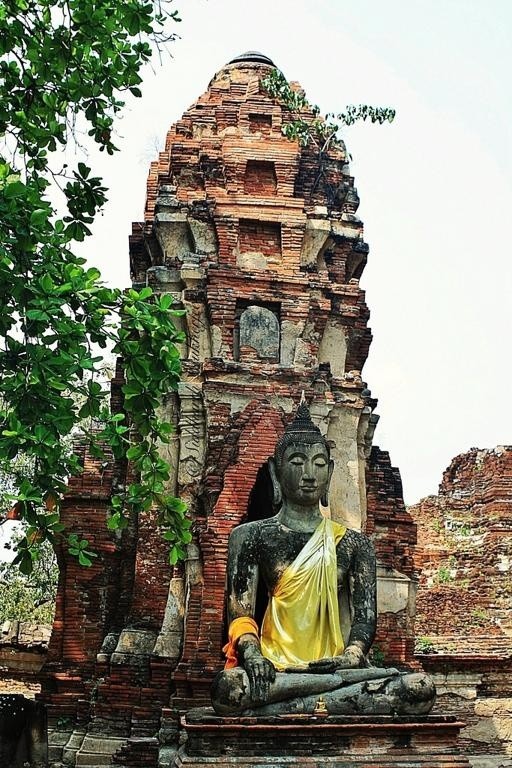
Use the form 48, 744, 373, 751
314, 697, 327, 712
211, 402, 436, 716
